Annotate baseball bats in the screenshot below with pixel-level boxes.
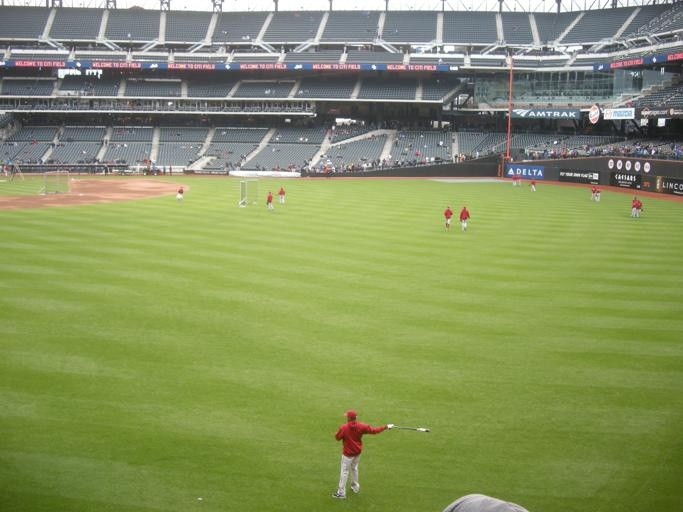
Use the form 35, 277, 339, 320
393, 426, 431, 435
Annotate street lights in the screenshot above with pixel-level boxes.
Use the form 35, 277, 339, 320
504, 56, 514, 160
221, 30, 228, 53
127, 33, 132, 54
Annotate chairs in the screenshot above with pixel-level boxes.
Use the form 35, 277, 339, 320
2, 4, 681, 171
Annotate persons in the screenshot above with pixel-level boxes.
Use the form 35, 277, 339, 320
595, 188, 600, 202
631, 197, 643, 218
444, 207, 453, 229
459, 206, 471, 231
177, 187, 184, 199
591, 186, 596, 200
266, 191, 274, 209
277, 187, 287, 205
529, 179, 537, 191
333, 411, 396, 497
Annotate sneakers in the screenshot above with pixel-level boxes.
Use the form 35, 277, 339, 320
351, 485, 359, 493
332, 493, 347, 499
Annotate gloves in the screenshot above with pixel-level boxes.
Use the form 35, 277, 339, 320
386, 422, 395, 430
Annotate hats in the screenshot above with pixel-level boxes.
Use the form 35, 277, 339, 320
344, 410, 358, 419
